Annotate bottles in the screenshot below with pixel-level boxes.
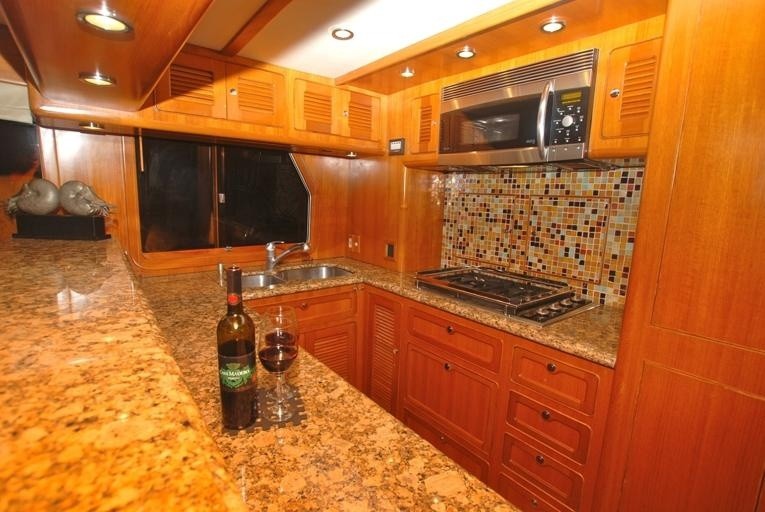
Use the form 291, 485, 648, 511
215, 265, 256, 429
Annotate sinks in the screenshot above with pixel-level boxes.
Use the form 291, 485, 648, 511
222, 270, 288, 289
274, 261, 356, 283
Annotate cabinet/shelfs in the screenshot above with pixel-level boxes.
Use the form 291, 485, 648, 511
141, 43, 290, 150
357, 286, 612, 512
243, 284, 366, 390
588, 16, 662, 167
402, 80, 439, 169
289, 70, 381, 160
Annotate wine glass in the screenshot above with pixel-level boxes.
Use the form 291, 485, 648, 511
257, 306, 298, 422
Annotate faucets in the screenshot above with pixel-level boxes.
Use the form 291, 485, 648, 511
264, 239, 312, 272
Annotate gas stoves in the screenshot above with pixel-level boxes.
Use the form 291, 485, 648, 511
412, 263, 599, 324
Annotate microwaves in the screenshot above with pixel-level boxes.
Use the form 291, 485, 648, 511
436, 46, 600, 175
